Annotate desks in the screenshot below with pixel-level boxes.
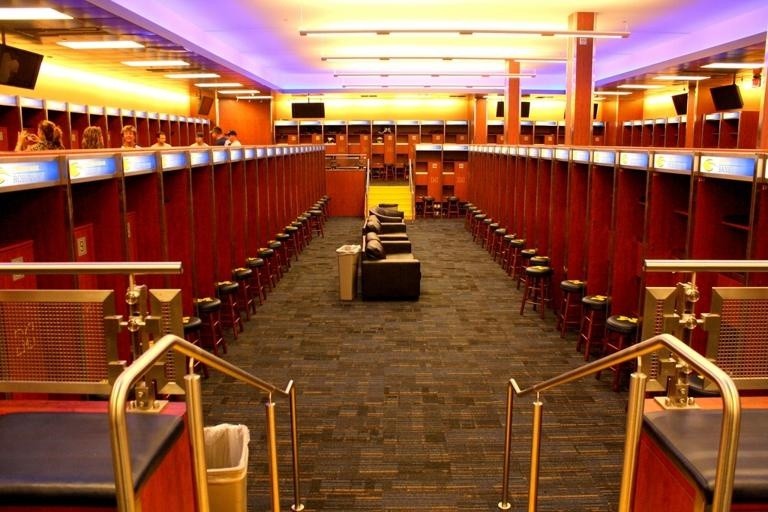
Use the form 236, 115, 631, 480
435, 201, 447, 218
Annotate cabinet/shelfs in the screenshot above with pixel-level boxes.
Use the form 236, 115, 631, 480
0, 96, 326, 397
273, 121, 473, 202
470, 111, 768, 398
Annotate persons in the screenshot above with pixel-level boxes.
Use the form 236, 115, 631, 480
14, 118, 66, 152
82, 125, 104, 149
190, 131, 210, 147
209, 126, 231, 146
149, 130, 173, 148
224, 130, 241, 147
120, 124, 141, 149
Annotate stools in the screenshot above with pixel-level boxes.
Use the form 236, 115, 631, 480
422, 196, 435, 218
463, 201, 640, 390
187, 192, 330, 386
448, 196, 460, 217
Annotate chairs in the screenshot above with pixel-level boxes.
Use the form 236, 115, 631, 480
358, 202, 420, 300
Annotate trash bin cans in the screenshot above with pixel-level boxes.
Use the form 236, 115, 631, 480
204, 423, 249, 512
336, 245, 361, 300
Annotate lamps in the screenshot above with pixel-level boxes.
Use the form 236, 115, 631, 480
297, 28, 632, 90
0, 7, 275, 104
592, 58, 763, 95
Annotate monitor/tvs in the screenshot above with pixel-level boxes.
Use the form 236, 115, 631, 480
197, 96, 213, 115
563, 103, 597, 120
291, 103, 325, 119
495, 102, 530, 117
672, 92, 688, 115
710, 84, 744, 109
0, 43, 44, 89
